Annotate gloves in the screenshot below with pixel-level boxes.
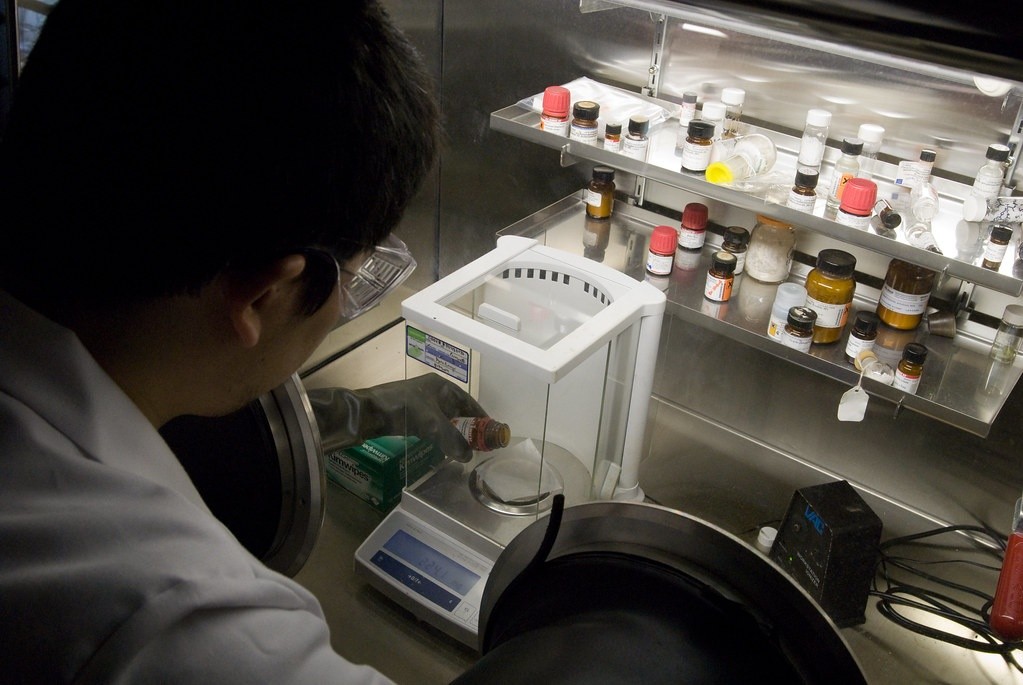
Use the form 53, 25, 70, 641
306, 372, 492, 464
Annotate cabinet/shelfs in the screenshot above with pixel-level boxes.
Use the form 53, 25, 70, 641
489, 77, 1023, 440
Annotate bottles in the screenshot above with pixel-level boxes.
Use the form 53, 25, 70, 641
450, 416, 511, 452
535, 86, 1023, 399
751, 526, 777, 554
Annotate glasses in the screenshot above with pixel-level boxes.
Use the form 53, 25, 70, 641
189, 227, 417, 323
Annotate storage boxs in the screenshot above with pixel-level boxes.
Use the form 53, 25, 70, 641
324, 434, 448, 513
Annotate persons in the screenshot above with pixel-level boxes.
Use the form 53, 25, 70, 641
0, 0, 492, 685
